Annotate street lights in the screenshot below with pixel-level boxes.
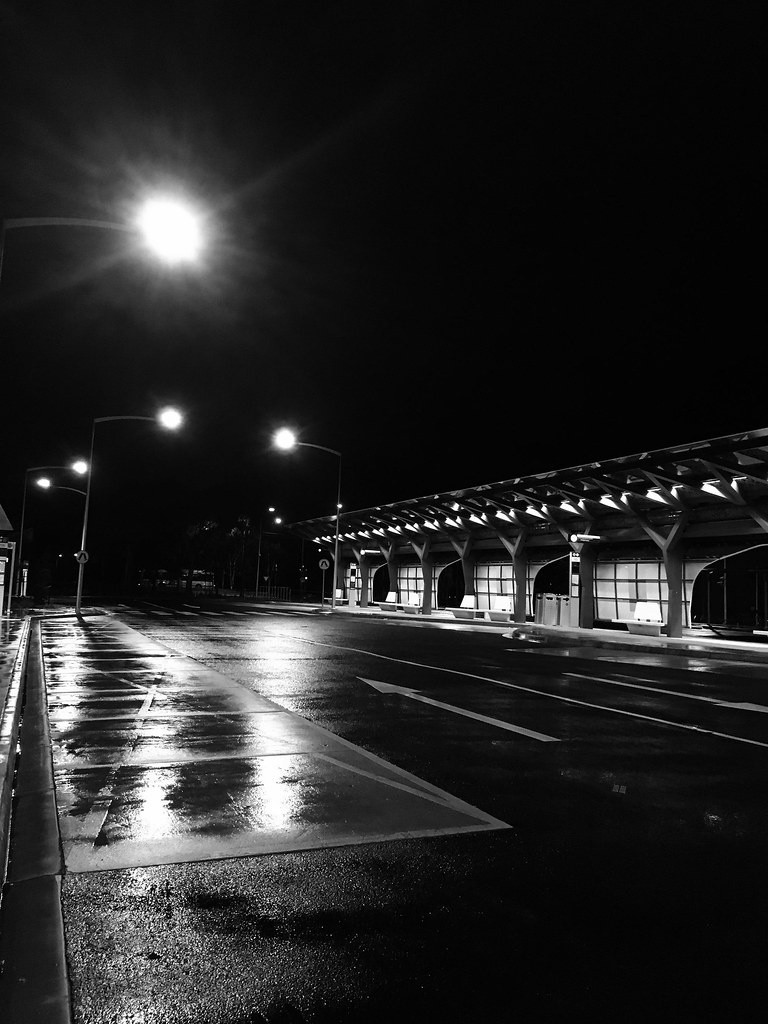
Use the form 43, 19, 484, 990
32, 407, 183, 615
270, 425, 343, 609
14, 460, 89, 598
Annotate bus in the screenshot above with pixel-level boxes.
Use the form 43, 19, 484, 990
136, 568, 218, 596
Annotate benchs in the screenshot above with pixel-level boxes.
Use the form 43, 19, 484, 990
612, 602, 667, 637
400, 594, 423, 615
482, 596, 514, 622
445, 595, 479, 620
370, 591, 401, 612
324, 589, 348, 606
